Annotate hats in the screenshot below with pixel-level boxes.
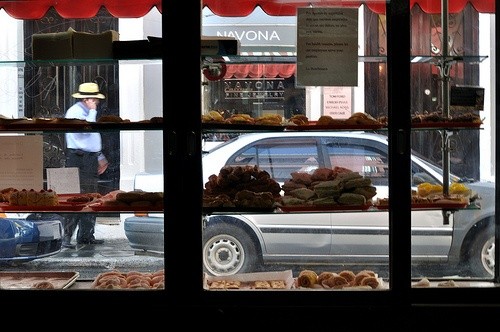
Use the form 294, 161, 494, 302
72, 83, 105, 99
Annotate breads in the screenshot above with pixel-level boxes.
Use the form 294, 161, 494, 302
0, 186, 163, 206
203, 111, 482, 128
416, 278, 455, 287
33, 280, 54, 289
34, 114, 164, 123
208, 269, 380, 289
95, 270, 165, 290
203, 163, 380, 208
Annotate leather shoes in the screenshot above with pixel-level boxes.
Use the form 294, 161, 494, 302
62, 243, 76, 249
79, 239, 104, 244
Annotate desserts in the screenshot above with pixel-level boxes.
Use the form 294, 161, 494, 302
418, 182, 467, 196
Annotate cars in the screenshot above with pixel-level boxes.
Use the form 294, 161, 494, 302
0, 212, 64, 267
118, 127, 495, 281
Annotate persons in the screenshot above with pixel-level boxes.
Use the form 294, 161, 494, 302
61, 83, 110, 249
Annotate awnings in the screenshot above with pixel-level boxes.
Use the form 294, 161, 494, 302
0, 0, 495, 19
203, 62, 297, 79
